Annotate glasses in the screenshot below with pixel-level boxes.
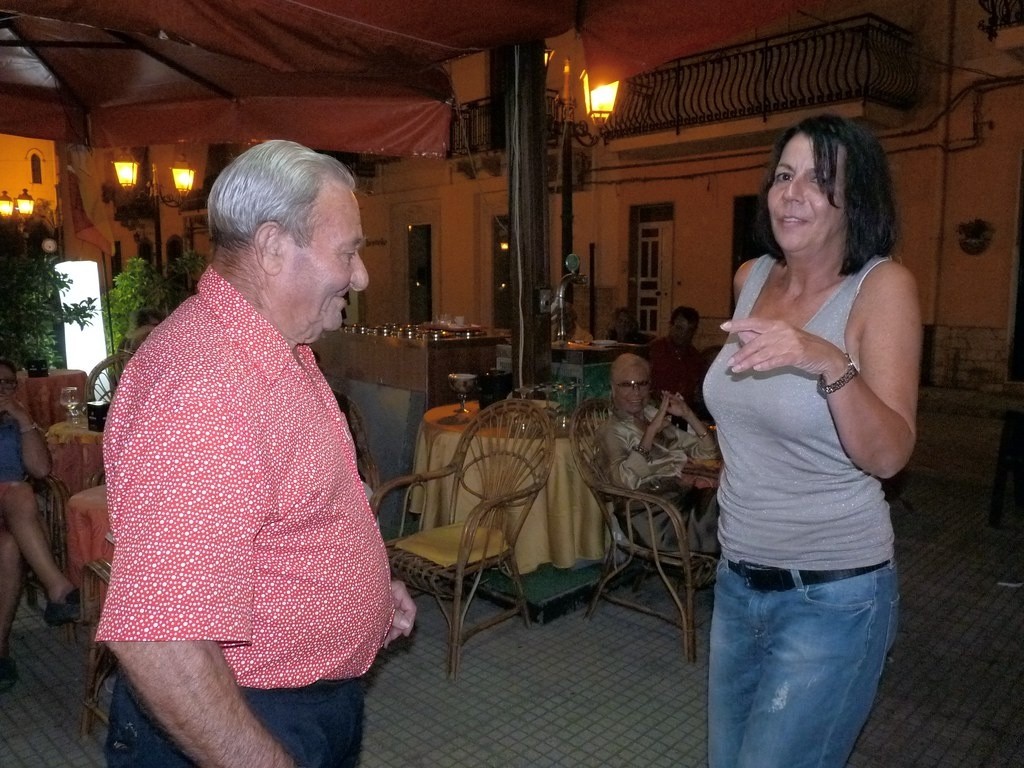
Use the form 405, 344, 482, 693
0, 379, 18, 390
615, 380, 649, 390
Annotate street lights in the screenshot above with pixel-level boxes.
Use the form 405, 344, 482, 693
544, 43, 623, 342
109, 144, 196, 285
0, 188, 36, 256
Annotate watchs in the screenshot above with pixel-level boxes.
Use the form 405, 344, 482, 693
819, 354, 858, 393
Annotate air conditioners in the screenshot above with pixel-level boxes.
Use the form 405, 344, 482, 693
546, 149, 583, 190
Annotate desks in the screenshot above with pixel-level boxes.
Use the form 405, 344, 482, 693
408, 404, 621, 578
45, 418, 110, 499
65, 485, 125, 634
15, 366, 96, 433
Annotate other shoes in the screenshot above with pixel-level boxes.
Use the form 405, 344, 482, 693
44, 589, 81, 625
0, 657, 18, 688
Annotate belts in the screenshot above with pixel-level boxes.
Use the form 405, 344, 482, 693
727, 560, 890, 591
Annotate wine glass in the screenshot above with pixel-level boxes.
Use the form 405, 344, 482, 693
60, 386, 80, 428
515, 380, 611, 435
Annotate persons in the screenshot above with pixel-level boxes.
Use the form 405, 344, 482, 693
596, 353, 721, 556
0, 358, 80, 645
98, 139, 417, 768
608, 306, 722, 433
703, 117, 923, 767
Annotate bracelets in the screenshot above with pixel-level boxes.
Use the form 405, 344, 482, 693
20, 421, 39, 434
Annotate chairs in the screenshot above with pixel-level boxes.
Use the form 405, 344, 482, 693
20, 353, 138, 740
330, 389, 389, 491
567, 400, 722, 665
364, 400, 557, 681
